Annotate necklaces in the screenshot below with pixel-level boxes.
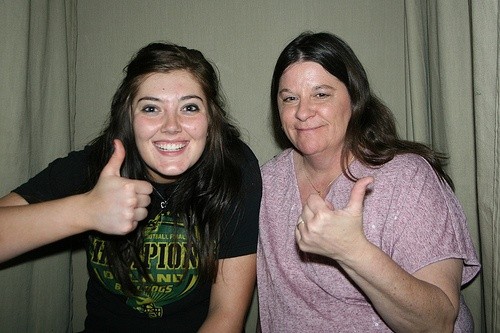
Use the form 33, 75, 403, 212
301, 156, 354, 196
151, 184, 180, 208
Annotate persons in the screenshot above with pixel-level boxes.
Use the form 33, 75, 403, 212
256, 29, 483, 333
0, 39, 261, 333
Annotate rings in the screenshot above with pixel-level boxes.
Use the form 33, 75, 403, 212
297, 221, 304, 229
